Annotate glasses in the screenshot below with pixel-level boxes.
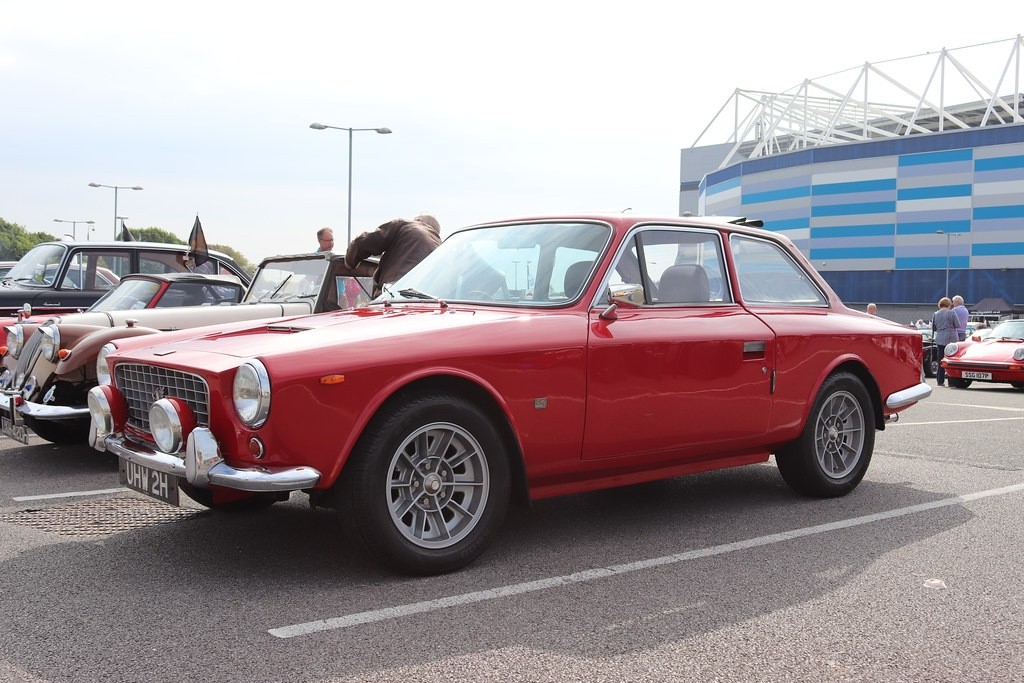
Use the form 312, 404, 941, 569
321, 239, 333, 242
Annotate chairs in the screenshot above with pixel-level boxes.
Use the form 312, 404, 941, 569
659, 265, 711, 304
159, 290, 185, 309
564, 261, 611, 305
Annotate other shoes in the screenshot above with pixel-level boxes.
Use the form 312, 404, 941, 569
938, 383, 946, 386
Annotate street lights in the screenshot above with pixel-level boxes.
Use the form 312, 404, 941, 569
935, 229, 962, 298
116, 216, 129, 277
512, 260, 532, 291
308, 123, 393, 248
53, 219, 96, 243
87, 182, 143, 273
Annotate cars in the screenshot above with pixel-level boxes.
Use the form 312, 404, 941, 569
4, 248, 410, 461
940, 318, 1024, 390
0, 259, 121, 287
87, 212, 933, 577
0, 273, 250, 380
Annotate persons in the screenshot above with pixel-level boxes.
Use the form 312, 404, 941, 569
866, 303, 877, 315
344, 215, 441, 302
311, 229, 359, 309
917, 295, 969, 387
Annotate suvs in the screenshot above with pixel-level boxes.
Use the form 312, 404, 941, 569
0, 239, 253, 320
919, 314, 1007, 379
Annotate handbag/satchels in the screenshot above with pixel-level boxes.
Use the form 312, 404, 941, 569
930, 348, 937, 363
